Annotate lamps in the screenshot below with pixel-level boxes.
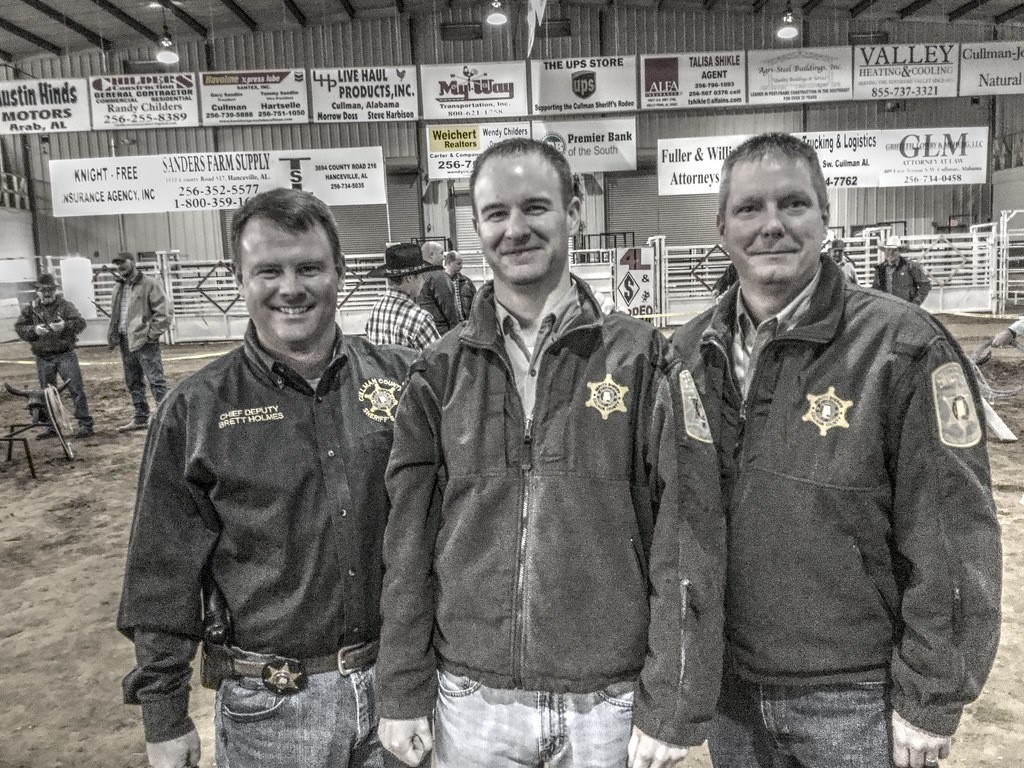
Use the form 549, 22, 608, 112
778, 0, 798, 38
157, 1, 179, 64
486, 0, 507, 25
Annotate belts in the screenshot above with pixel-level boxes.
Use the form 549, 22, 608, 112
230, 639, 380, 678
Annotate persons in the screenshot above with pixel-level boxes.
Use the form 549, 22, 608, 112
666, 133, 1002, 768
107, 252, 174, 432
830, 239, 859, 285
15, 274, 96, 438
365, 241, 476, 350
114, 187, 422, 768
873, 235, 932, 306
711, 262, 738, 298
992, 319, 1024, 349
376, 140, 725, 768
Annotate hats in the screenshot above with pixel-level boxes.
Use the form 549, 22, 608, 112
28, 274, 60, 288
112, 252, 134, 264
828, 239, 847, 250
365, 243, 444, 278
878, 236, 910, 253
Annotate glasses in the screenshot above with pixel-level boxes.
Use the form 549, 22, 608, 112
38, 287, 56, 293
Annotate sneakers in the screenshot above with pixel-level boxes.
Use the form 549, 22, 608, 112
75, 425, 94, 438
35, 426, 59, 440
119, 419, 149, 433
